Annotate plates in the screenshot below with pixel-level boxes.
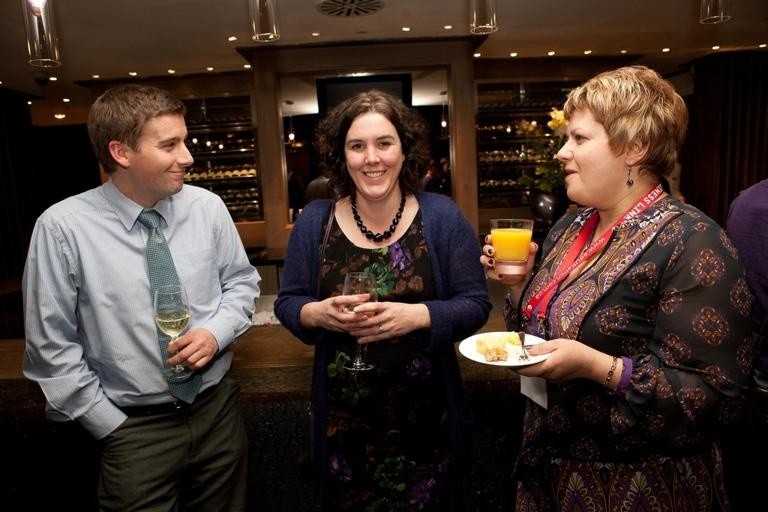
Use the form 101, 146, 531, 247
458, 331, 553, 367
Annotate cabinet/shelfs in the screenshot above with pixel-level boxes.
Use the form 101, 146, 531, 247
476, 107, 562, 196
183, 120, 263, 220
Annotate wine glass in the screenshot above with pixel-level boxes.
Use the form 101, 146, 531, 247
341, 271, 377, 371
153, 284, 194, 381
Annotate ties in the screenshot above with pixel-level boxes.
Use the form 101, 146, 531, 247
138, 210, 203, 406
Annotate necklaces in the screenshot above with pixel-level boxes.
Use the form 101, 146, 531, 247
347, 192, 407, 242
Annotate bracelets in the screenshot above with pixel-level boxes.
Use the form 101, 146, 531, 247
602, 356, 617, 387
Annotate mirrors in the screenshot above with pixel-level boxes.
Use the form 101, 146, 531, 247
271, 63, 455, 233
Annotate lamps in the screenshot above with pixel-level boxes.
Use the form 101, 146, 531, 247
468, 0, 499, 35
21, 0, 62, 68
699, 0, 732, 25
247, 0, 280, 42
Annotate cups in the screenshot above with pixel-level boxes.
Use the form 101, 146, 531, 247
489, 218, 533, 276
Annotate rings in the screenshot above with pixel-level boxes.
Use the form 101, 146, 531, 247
377, 324, 385, 334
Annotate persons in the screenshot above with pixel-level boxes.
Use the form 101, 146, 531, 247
726, 179, 768, 512
274, 90, 493, 511
480, 65, 753, 512
416, 155, 452, 194
22, 84, 261, 511
287, 160, 340, 221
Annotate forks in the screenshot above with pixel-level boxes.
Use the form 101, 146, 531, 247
517, 332, 531, 363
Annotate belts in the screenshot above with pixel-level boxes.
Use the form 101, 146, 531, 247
121, 387, 218, 417
